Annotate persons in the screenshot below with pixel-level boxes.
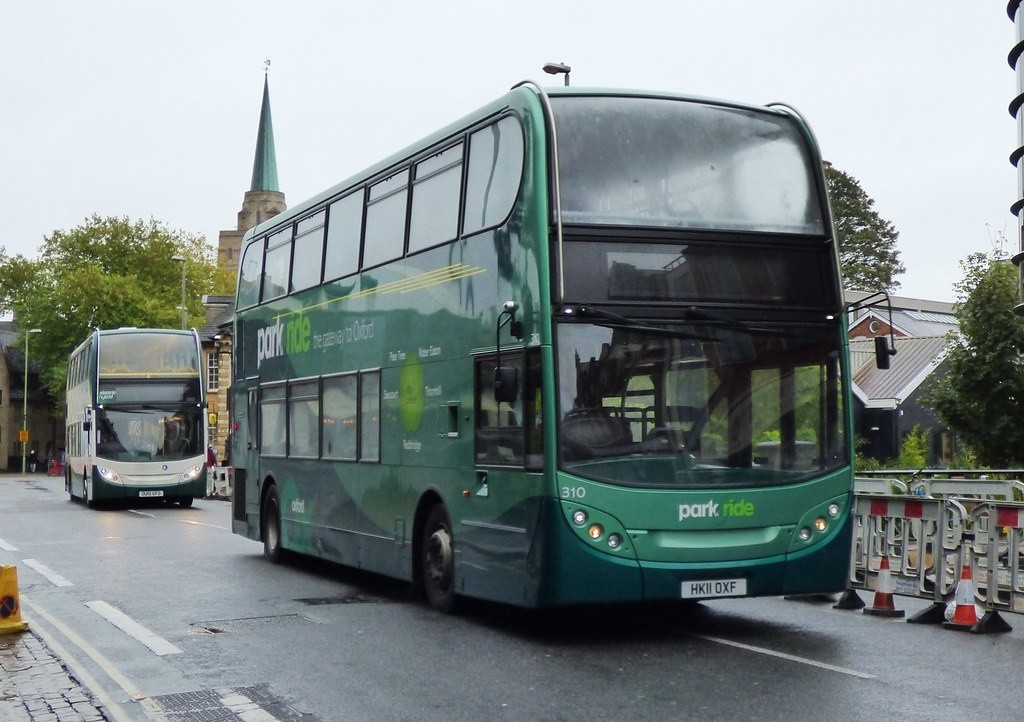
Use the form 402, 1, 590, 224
28, 450, 39, 472
50, 450, 65, 476
514, 355, 588, 465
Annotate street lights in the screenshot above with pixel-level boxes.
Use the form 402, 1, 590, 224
172, 256, 187, 329
22, 328, 42, 475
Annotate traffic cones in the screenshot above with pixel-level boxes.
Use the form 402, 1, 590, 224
863, 556, 906, 618
942, 564, 979, 630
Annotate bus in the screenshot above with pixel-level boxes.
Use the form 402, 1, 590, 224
228, 80, 899, 614
63, 326, 218, 510
